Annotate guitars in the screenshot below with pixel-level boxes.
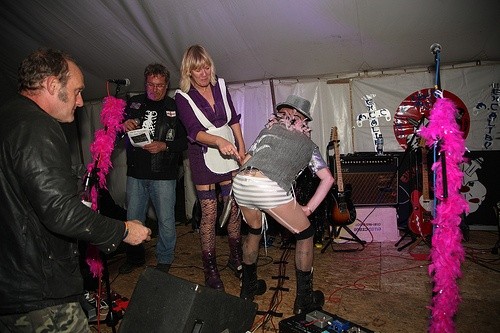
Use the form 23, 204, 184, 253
329, 125, 357, 226
407, 136, 437, 236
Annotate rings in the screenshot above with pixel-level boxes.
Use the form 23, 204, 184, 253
225, 151, 228, 153
228, 149, 231, 152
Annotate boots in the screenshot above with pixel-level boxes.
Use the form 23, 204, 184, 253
239, 260, 267, 303
294, 268, 325, 314
204, 259, 225, 293
227, 242, 243, 278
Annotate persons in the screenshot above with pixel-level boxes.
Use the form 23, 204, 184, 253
0, 47, 152, 333
231, 94, 335, 315
173, 45, 247, 292
119, 64, 188, 274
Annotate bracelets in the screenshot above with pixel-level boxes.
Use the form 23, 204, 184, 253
306, 205, 312, 213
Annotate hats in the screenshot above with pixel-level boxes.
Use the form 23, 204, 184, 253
276, 97, 314, 122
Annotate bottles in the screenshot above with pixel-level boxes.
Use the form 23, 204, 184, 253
377, 134, 383, 156
244, 166, 256, 176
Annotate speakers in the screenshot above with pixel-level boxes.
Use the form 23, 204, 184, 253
117, 264, 258, 333
332, 156, 399, 205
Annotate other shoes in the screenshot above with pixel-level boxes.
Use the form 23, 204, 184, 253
118, 243, 146, 274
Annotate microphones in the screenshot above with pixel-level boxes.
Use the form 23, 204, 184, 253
107, 79, 130, 87
